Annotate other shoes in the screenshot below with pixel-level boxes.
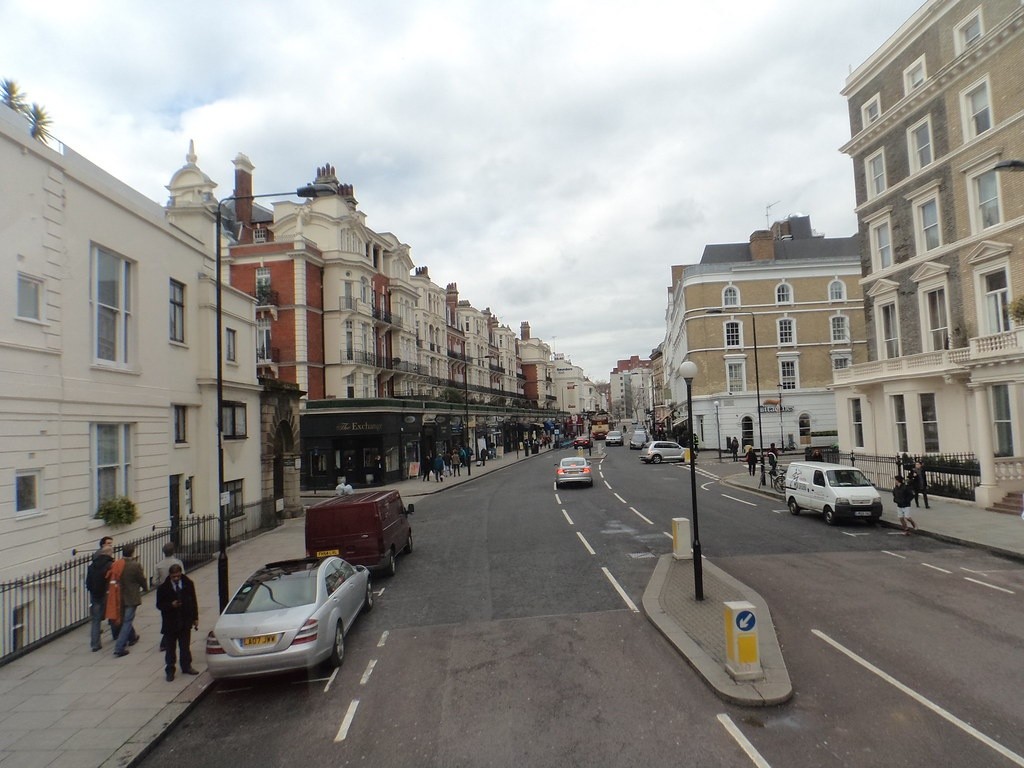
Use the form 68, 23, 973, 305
113, 635, 118, 639
904, 527, 910, 534
114, 649, 129, 656
916, 502, 919, 507
926, 506, 930, 509
129, 635, 140, 645
913, 524, 917, 530
92, 645, 102, 652
182, 668, 199, 674
440, 476, 443, 482
166, 672, 175, 681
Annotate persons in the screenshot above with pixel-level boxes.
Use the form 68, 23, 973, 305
86, 536, 148, 657
652, 427, 668, 441
156, 563, 199, 682
694, 433, 700, 453
744, 442, 758, 476
892, 475, 918, 532
523, 435, 552, 449
152, 544, 185, 650
459, 442, 473, 467
911, 461, 929, 509
731, 437, 739, 462
766, 443, 780, 470
812, 450, 824, 462
434, 454, 444, 483
374, 455, 384, 482
451, 449, 461, 477
442, 450, 453, 477
481, 447, 489, 466
422, 455, 432, 481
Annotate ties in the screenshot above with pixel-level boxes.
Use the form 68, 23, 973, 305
175, 582, 182, 594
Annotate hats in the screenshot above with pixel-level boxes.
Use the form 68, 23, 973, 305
438, 454, 443, 457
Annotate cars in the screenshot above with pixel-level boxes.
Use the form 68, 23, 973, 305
554, 458, 593, 489
206, 556, 374, 678
629, 426, 649, 450
632, 419, 638, 424
604, 431, 624, 446
574, 436, 593, 449
638, 441, 698, 464
594, 429, 606, 440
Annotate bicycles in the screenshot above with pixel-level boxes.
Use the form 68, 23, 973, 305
774, 465, 787, 494
776, 439, 798, 452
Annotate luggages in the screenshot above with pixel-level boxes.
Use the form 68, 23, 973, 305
476, 461, 482, 466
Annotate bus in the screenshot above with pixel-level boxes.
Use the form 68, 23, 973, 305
590, 412, 613, 438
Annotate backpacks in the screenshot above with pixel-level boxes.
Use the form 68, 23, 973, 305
87, 556, 111, 592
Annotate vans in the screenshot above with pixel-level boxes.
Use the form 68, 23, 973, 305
785, 461, 883, 526
305, 489, 415, 577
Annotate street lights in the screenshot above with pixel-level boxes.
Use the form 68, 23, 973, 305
706, 308, 766, 485
215, 185, 339, 616
561, 384, 579, 426
679, 362, 704, 600
777, 382, 785, 453
464, 355, 494, 475
714, 401, 721, 459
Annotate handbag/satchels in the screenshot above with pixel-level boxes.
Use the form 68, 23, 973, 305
894, 497, 904, 504
746, 453, 750, 461
485, 456, 488, 460
907, 470, 916, 484
